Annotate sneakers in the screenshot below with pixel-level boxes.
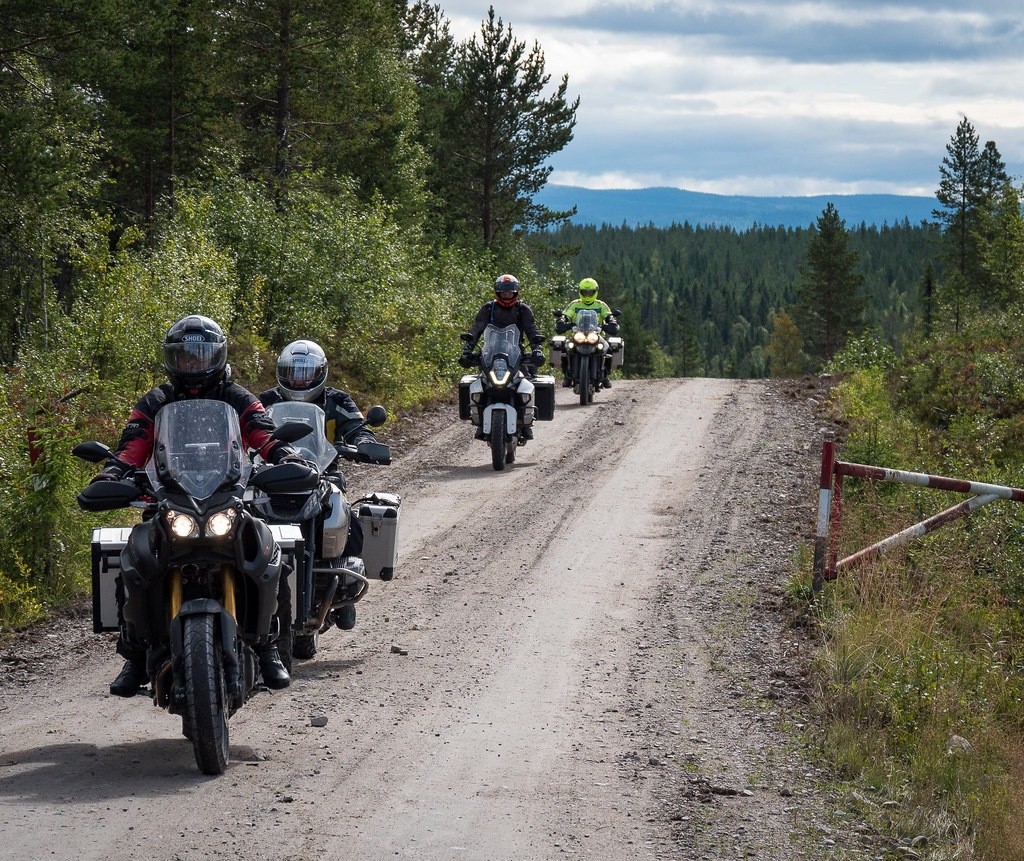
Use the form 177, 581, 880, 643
334, 602, 356, 630
110, 659, 151, 698
258, 645, 291, 690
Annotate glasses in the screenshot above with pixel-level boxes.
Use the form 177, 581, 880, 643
286, 374, 314, 383
176, 350, 214, 362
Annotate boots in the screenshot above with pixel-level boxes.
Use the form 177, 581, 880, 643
602, 358, 612, 388
561, 357, 572, 387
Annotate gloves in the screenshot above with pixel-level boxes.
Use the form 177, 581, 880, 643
605, 315, 617, 336
354, 432, 380, 465
89, 465, 124, 485
556, 315, 569, 334
533, 349, 546, 367
271, 447, 309, 468
459, 352, 473, 367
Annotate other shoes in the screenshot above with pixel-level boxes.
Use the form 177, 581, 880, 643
522, 427, 533, 439
475, 427, 484, 440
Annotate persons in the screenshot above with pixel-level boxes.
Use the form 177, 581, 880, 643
256, 340, 378, 630
555, 278, 619, 388
461, 274, 544, 440
88, 315, 310, 697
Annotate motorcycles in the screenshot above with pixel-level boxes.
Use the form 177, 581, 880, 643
72, 400, 314, 776
247, 402, 401, 658
548, 308, 624, 405
459, 324, 555, 470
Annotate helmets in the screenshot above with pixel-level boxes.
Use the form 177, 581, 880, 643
276, 340, 328, 402
579, 278, 599, 304
162, 315, 228, 399
494, 275, 519, 307
